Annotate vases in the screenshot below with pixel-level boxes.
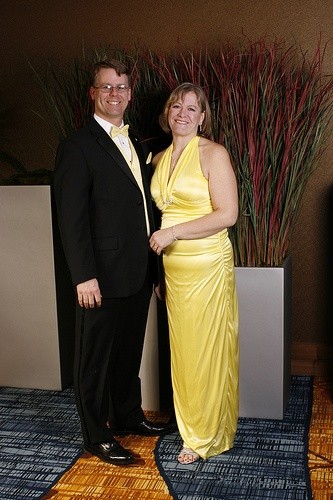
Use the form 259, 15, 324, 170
139, 254, 293, 420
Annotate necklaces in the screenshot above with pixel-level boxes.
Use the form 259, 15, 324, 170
172, 143, 186, 169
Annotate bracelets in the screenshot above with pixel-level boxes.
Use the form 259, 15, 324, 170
172, 225, 178, 241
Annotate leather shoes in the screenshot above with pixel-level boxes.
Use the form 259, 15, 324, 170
110, 418, 169, 436
83, 434, 134, 464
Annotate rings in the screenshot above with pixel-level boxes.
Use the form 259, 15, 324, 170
153, 247, 157, 251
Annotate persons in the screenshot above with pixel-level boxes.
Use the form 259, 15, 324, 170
52, 60, 167, 464
148, 83, 239, 465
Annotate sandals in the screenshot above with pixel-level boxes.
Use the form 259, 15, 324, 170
178, 447, 200, 465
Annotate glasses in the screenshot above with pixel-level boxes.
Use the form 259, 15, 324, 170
94, 84, 132, 93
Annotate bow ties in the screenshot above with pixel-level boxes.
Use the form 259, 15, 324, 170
111, 124, 129, 138
